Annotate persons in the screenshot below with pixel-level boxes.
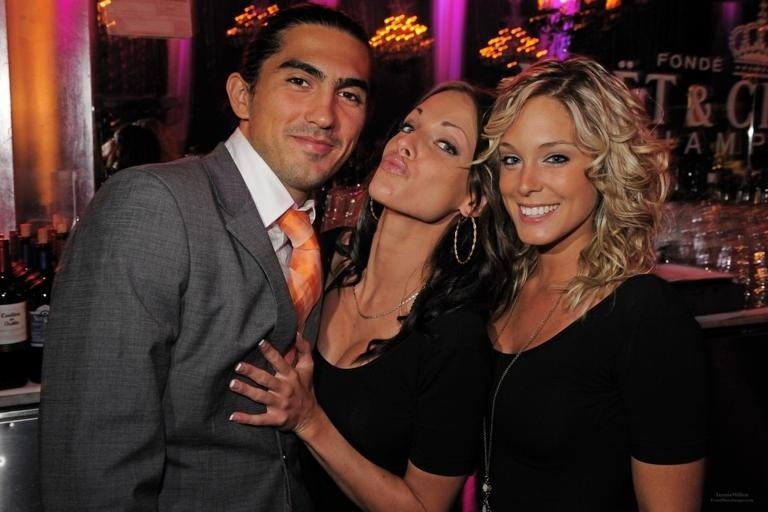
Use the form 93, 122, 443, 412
34, 4, 371, 512
94, 103, 166, 176
228, 79, 496, 512
454, 53, 717, 512
359, 126, 386, 163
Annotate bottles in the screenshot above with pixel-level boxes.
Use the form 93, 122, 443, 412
0, 215, 70, 393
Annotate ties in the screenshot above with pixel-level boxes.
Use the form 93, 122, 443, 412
277, 208, 323, 365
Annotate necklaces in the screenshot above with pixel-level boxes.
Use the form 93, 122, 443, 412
348, 284, 420, 319
479, 288, 563, 512
